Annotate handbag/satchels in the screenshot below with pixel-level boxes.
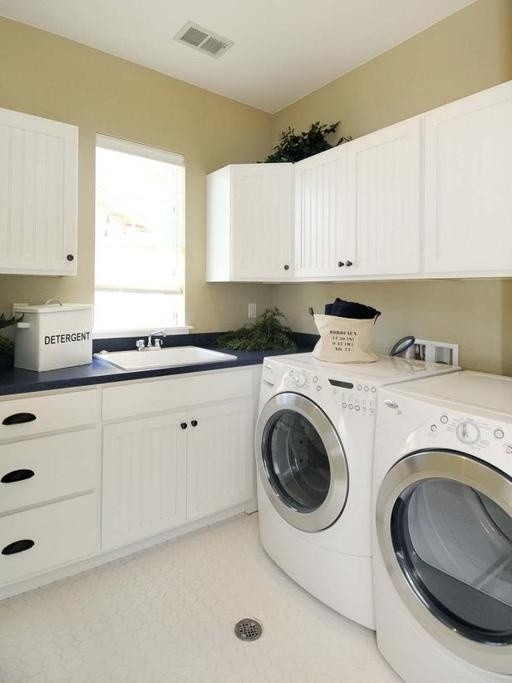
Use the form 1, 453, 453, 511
309, 307, 380, 363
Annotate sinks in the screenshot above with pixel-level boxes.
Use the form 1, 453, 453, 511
93, 345, 238, 372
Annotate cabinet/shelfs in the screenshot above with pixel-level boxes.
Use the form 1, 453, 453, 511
0, 382, 96, 601
295, 75, 511, 287
0, 106, 83, 280
202, 161, 296, 283
98, 360, 256, 559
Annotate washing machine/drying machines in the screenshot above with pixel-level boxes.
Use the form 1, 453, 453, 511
375, 369, 512, 683
254, 352, 462, 632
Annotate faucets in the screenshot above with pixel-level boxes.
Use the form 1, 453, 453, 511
147, 330, 167, 347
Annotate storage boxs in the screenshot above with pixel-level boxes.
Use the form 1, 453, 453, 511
11, 296, 95, 370
